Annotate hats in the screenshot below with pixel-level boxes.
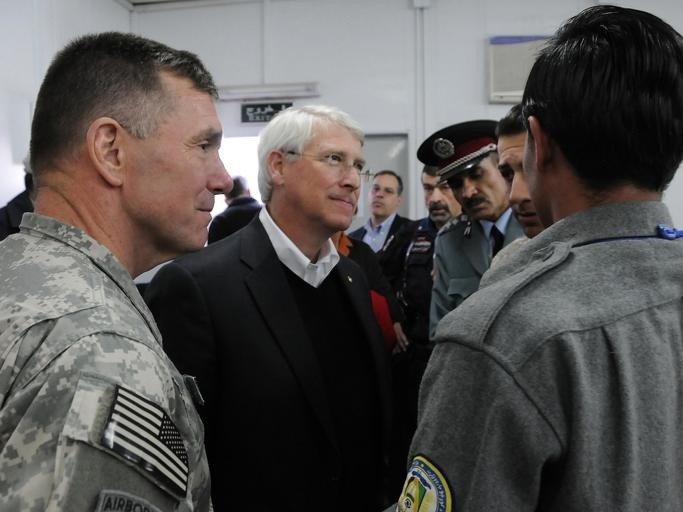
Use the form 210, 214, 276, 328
417, 120, 500, 184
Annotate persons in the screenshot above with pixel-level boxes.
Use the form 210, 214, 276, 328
414, 118, 525, 349
204, 172, 264, 246
330, 229, 410, 362
391, 163, 462, 437
346, 169, 414, 260
140, 101, 407, 510
381, 4, 683, 510
0, 148, 37, 243
492, 101, 546, 240
0, 31, 235, 512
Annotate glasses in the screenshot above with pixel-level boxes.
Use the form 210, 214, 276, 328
289, 149, 371, 182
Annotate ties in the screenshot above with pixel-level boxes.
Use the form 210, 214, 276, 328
490, 228, 503, 257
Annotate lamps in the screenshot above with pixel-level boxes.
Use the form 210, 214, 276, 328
214, 81, 320, 101
